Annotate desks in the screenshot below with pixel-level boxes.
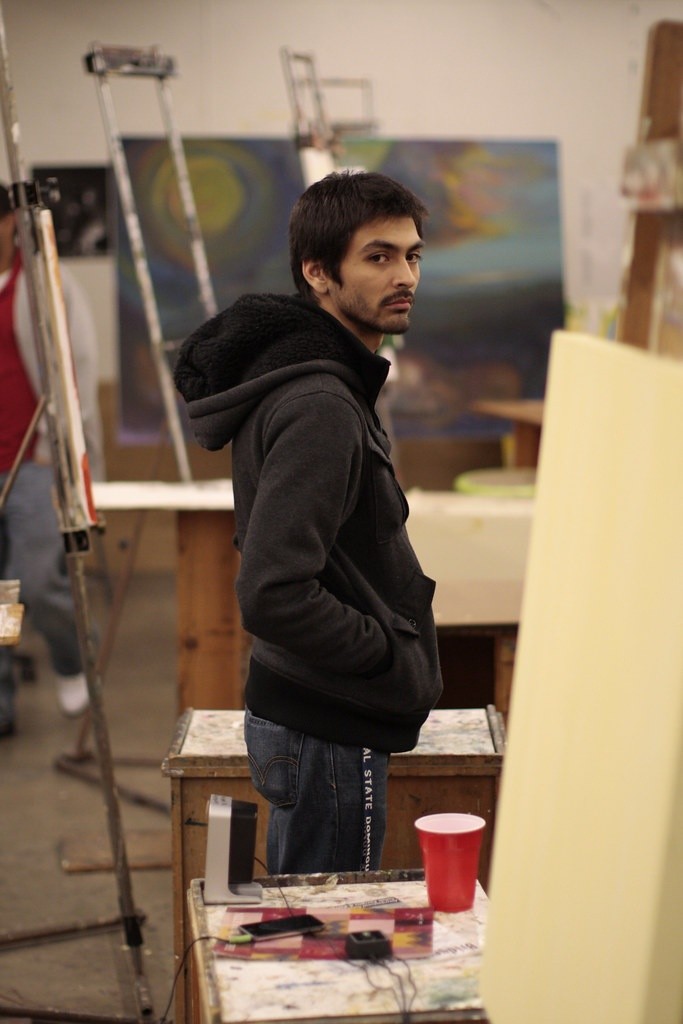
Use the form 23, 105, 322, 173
163, 401, 545, 1024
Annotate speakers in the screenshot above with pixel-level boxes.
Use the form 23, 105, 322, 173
202, 794, 263, 904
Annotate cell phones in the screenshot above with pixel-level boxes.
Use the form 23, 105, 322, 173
239, 914, 325, 942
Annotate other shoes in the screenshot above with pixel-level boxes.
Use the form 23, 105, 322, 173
51, 672, 91, 718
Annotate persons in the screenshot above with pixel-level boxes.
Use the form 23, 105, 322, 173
172, 169, 446, 877
0, 185, 91, 713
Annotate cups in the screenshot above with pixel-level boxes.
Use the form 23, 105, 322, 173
414, 812, 485, 912
